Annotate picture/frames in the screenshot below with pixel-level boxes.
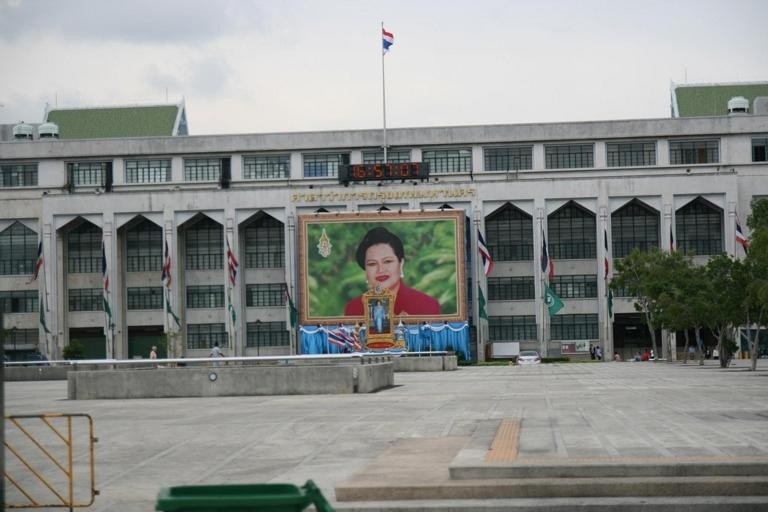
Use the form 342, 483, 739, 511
363, 284, 395, 339
298, 209, 465, 326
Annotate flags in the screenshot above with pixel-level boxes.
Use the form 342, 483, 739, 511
383, 30, 393, 56
328, 330, 354, 351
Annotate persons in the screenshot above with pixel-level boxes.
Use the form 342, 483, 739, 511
705, 346, 711, 360
353, 325, 361, 352
149, 346, 158, 368
208, 342, 225, 366
713, 347, 719, 360
589, 344, 649, 361
689, 345, 696, 360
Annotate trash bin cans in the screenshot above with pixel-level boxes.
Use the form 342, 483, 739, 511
154, 478, 335, 512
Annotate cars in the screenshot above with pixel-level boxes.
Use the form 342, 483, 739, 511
514, 351, 542, 365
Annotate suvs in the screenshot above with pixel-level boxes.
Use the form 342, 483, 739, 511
26, 353, 45, 367
705, 345, 719, 360
